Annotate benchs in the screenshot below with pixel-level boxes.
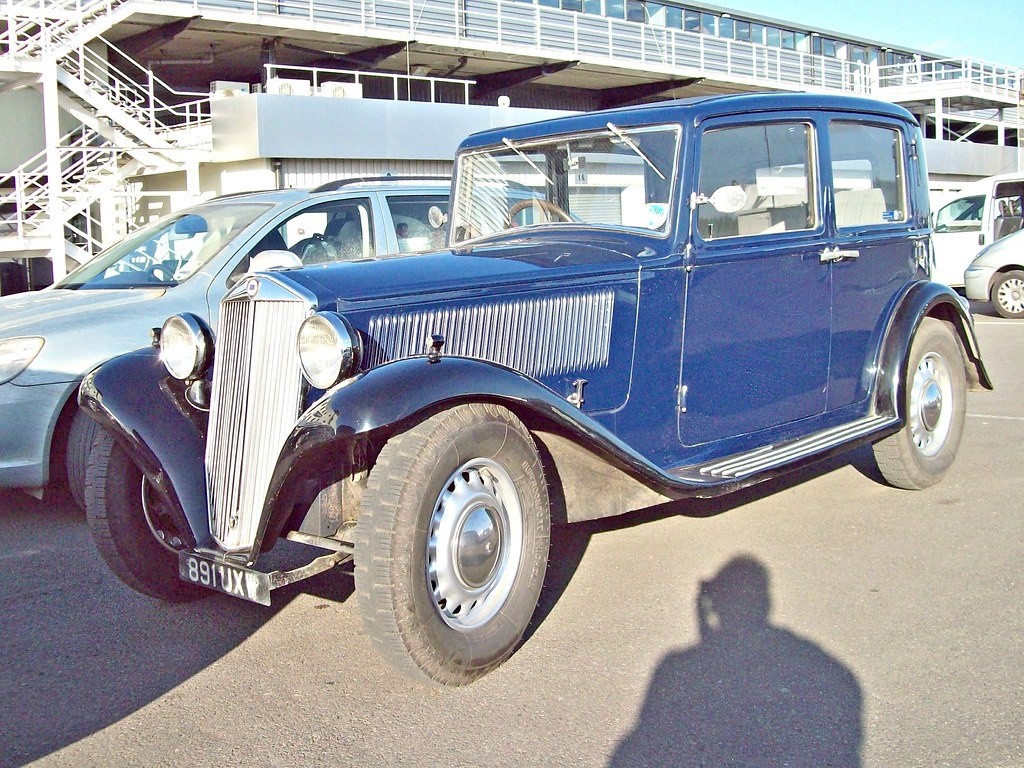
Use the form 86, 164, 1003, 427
995, 199, 1023, 241
693, 184, 893, 235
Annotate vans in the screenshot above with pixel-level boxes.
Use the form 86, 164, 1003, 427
932, 171, 1024, 289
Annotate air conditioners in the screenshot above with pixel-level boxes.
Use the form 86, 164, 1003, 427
211, 80, 249, 97
321, 82, 362, 98
266, 78, 310, 96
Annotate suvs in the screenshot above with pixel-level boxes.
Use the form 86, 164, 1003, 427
77, 92, 1001, 689
1, 169, 590, 514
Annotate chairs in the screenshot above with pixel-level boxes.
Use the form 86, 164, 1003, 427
306, 216, 363, 265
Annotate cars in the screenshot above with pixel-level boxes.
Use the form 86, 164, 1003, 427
963, 226, 1024, 320
